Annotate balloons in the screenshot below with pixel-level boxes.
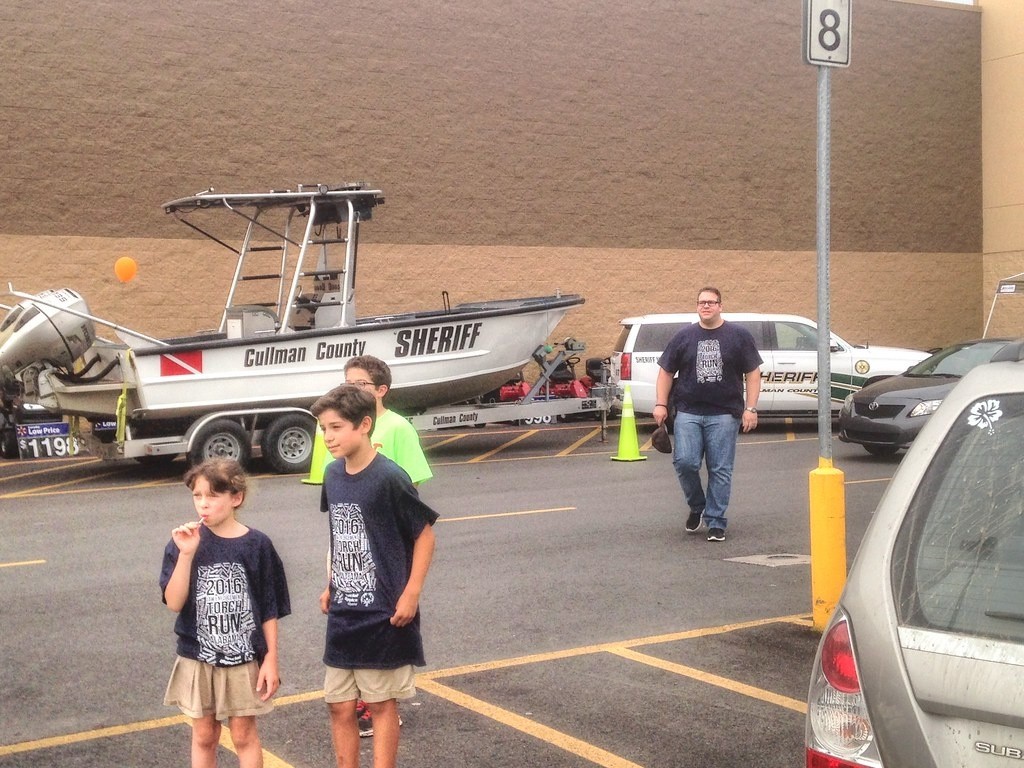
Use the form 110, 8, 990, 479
115, 257, 136, 283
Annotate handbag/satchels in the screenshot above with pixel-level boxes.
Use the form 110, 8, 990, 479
663, 378, 678, 436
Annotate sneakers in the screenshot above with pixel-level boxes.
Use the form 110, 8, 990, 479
685, 499, 706, 532
707, 528, 726, 541
356, 698, 403, 738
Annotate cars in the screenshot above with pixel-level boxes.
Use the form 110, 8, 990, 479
838, 337, 1024, 454
803, 362, 1024, 768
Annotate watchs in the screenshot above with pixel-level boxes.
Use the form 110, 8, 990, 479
746, 407, 757, 413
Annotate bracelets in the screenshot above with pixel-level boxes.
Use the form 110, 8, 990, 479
656, 404, 667, 409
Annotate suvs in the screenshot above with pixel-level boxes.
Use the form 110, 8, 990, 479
611, 313, 936, 436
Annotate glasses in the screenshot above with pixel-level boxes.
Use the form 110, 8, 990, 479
339, 381, 376, 391
697, 300, 721, 307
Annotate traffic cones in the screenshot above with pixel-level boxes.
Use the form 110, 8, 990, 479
608, 384, 649, 462
303, 410, 335, 490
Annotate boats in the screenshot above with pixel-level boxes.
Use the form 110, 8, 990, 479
0, 179, 587, 427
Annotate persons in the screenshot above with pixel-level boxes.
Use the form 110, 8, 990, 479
310, 356, 440, 768
160, 457, 292, 768
653, 287, 764, 542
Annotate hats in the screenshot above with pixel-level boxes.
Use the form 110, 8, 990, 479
651, 420, 672, 454
